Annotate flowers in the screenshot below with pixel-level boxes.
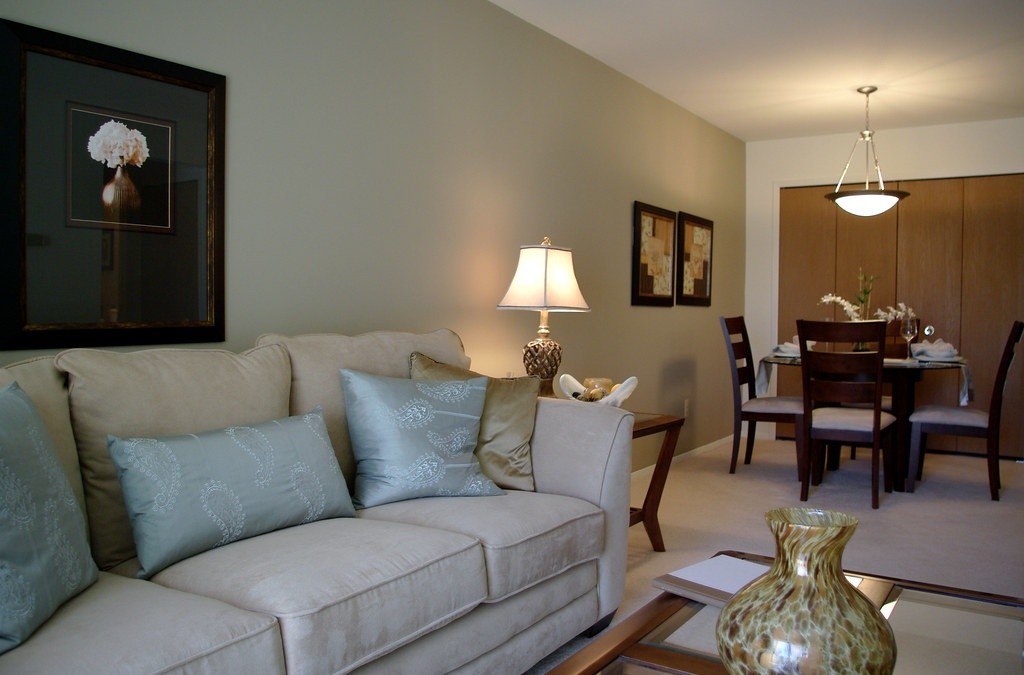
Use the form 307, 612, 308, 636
812, 265, 917, 323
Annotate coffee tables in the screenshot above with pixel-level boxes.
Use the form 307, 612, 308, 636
542, 549, 1024, 675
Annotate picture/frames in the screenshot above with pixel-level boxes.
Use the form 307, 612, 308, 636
630, 199, 678, 308
675, 210, 713, 308
0, 16, 227, 353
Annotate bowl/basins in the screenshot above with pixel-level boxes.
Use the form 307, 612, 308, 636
559, 373, 638, 407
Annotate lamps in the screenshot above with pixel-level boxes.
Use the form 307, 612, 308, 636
496, 236, 592, 398
824, 85, 911, 217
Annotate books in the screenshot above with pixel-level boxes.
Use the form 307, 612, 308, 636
652, 546, 893, 617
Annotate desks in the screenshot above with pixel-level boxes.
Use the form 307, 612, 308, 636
754, 355, 976, 493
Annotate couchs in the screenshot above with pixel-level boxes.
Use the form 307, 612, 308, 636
0, 326, 635, 675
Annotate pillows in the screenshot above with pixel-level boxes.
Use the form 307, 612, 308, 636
336, 366, 508, 512
408, 349, 542, 492
106, 404, 361, 581
0, 381, 100, 656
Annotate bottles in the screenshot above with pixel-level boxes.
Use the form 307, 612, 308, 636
715, 508, 897, 675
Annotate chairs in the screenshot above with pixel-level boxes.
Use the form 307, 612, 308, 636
833, 318, 920, 471
719, 315, 804, 482
795, 318, 897, 511
905, 320, 1024, 501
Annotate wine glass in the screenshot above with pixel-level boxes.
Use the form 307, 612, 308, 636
900, 317, 917, 361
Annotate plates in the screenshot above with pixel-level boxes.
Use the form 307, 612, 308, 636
771, 351, 800, 358
926, 351, 957, 357
917, 357, 959, 361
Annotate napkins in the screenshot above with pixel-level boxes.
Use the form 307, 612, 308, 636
771, 342, 801, 354
910, 343, 958, 359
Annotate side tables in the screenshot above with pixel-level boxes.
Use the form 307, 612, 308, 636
629, 410, 686, 553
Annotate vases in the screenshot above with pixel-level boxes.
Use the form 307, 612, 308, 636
851, 342, 871, 352
715, 507, 899, 675
